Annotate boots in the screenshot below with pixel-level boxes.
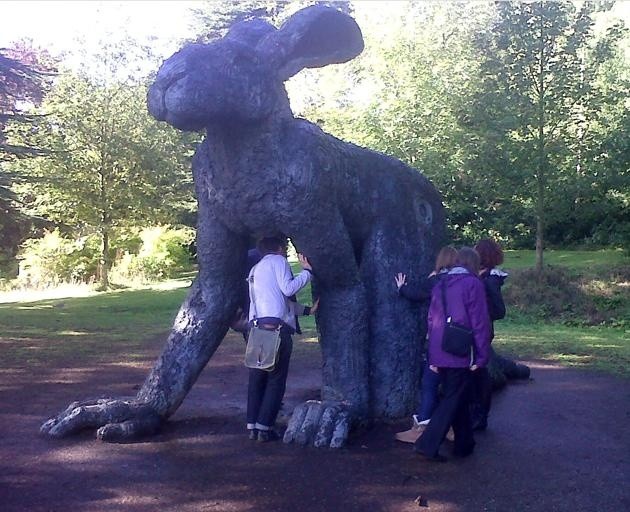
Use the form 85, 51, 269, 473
395, 415, 431, 443
446, 425, 454, 441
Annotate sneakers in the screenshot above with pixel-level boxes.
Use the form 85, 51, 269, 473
248, 429, 282, 442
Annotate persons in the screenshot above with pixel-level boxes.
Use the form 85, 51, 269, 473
242, 235, 320, 445
471, 238, 509, 434
409, 246, 492, 460
393, 244, 460, 445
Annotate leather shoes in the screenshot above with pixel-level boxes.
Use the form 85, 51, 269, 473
413, 445, 447, 461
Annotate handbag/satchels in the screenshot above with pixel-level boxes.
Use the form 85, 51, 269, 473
441, 321, 475, 357
244, 326, 281, 372
